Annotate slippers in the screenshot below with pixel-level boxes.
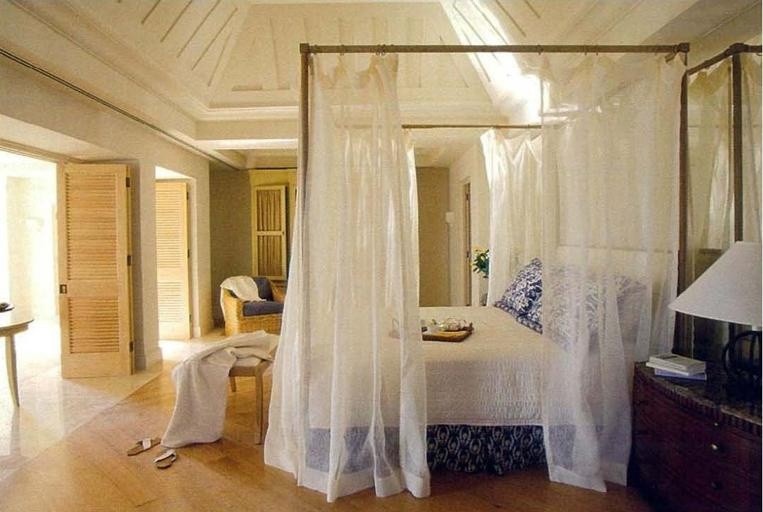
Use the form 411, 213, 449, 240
154, 449, 176, 468
127, 437, 160, 456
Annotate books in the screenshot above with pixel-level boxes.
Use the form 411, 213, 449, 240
644, 351, 710, 381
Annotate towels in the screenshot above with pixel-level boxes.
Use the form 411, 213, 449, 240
160, 330, 274, 448
221, 276, 267, 302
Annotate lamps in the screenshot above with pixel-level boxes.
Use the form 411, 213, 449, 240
666, 238, 762, 395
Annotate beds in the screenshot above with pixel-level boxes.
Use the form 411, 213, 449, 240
264, 42, 690, 471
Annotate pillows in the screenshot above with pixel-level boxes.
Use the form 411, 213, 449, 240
521, 272, 656, 351
494, 256, 589, 318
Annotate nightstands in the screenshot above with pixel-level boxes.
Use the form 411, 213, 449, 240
628, 359, 762, 512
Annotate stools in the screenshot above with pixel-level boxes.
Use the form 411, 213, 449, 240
195, 336, 279, 444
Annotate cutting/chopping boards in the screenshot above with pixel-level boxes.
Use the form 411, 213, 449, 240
390, 325, 472, 342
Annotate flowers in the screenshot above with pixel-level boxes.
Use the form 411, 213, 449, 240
470, 242, 490, 278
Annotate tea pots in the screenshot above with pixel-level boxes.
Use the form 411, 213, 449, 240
431, 316, 467, 331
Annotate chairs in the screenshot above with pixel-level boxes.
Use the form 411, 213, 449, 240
220, 276, 286, 337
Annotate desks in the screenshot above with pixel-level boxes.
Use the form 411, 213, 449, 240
0, 316, 34, 407
